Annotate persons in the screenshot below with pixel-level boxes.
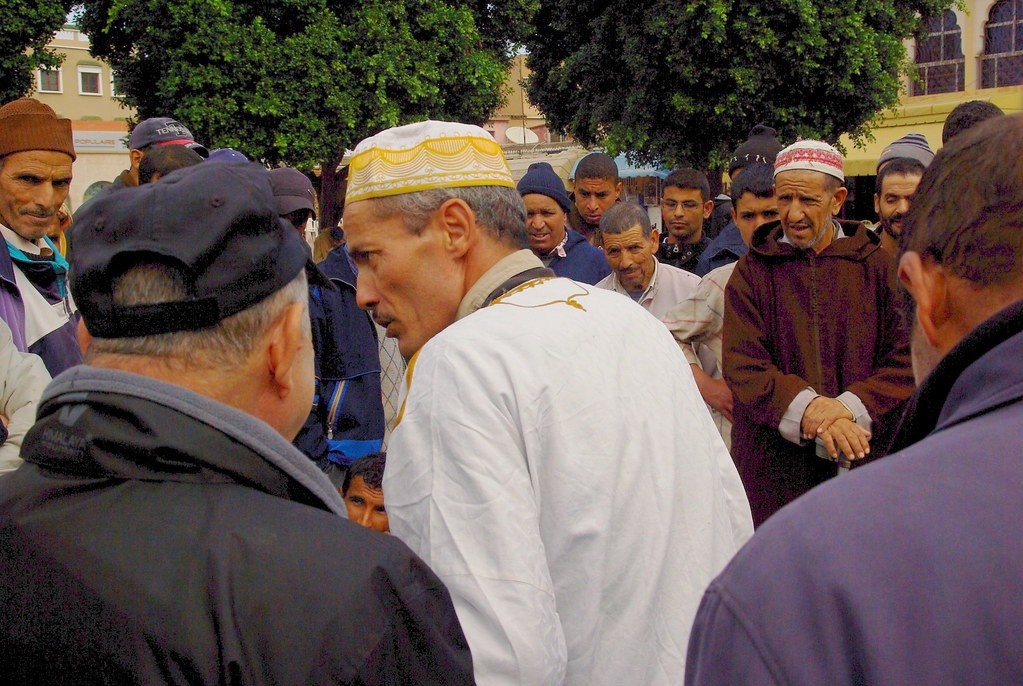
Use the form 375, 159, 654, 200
683, 100, 1022, 685
0, 96, 756, 686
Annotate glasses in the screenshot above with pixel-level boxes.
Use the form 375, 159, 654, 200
660, 197, 705, 211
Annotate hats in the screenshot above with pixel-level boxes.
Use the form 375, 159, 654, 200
204, 147, 248, 162
0, 98, 77, 164
129, 117, 210, 160
342, 119, 518, 205
773, 141, 846, 186
515, 163, 572, 213
877, 133, 935, 169
66, 160, 310, 339
728, 125, 783, 180
266, 167, 317, 222
